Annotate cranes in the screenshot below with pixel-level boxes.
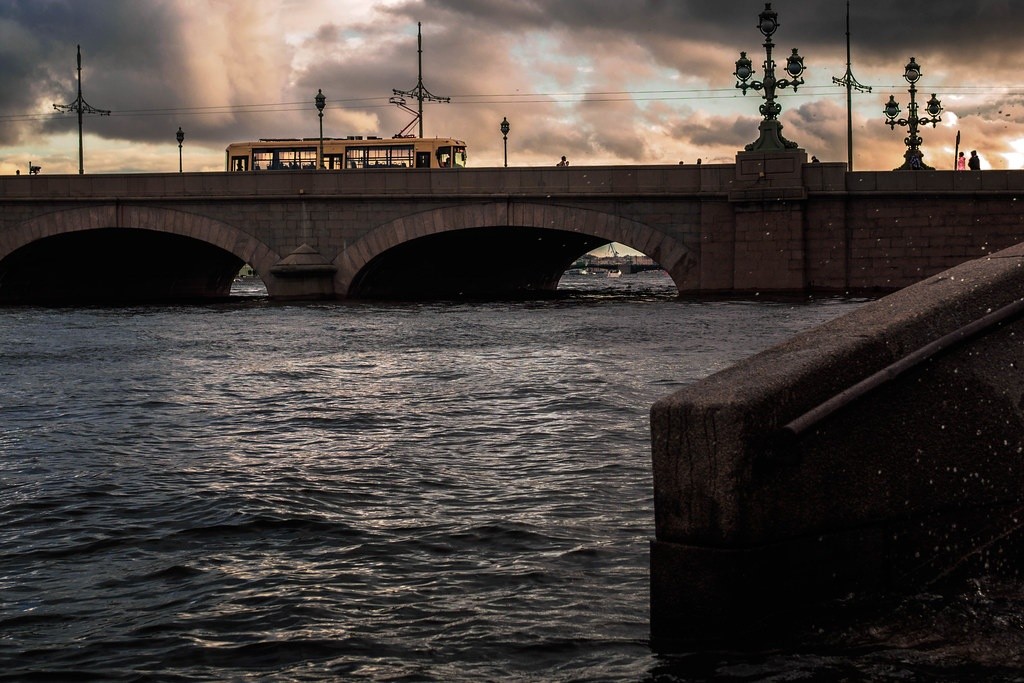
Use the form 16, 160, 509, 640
609, 243, 619, 257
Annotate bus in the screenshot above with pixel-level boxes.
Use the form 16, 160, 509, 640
225, 136, 467, 168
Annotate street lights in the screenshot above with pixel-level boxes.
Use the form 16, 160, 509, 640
314, 88, 326, 167
500, 116, 509, 167
881, 56, 942, 170
733, 0, 805, 149
176, 127, 185, 173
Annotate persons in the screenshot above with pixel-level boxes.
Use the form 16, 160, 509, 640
811, 155, 820, 163
557, 156, 566, 167
958, 152, 966, 170
566, 161, 570, 166
968, 150, 980, 170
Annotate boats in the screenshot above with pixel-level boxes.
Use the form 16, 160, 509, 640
607, 269, 622, 278
564, 269, 587, 274
